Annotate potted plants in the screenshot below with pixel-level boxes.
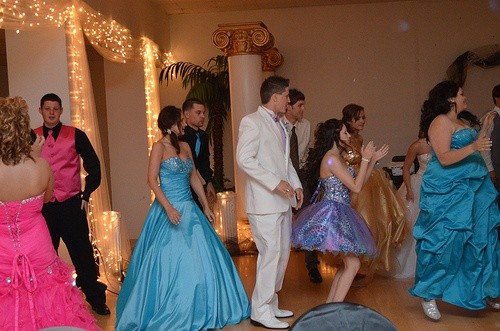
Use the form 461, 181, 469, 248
158, 55, 237, 251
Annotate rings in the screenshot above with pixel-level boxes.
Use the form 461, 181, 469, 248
286, 189, 290, 192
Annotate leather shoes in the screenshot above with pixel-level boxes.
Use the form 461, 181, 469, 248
274, 309, 294, 318
92, 304, 110, 315
250, 316, 290, 329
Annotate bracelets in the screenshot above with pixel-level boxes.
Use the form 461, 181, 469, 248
362, 157, 370, 162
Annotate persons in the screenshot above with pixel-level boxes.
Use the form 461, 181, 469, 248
30, 93, 111, 315
320, 103, 412, 288
408, 81, 500, 320
383, 128, 433, 279
478, 84, 500, 211
115, 105, 251, 331
0, 96, 101, 331
281, 88, 310, 171
290, 117, 390, 302
306, 117, 340, 283
236, 76, 304, 329
178, 98, 217, 213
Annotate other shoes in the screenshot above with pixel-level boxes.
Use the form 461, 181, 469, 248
354, 273, 365, 281
308, 268, 322, 282
421, 299, 441, 320
488, 302, 500, 309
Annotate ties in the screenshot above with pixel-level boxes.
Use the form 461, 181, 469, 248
195, 133, 201, 158
46, 130, 55, 152
290, 126, 299, 171
273, 116, 286, 154
